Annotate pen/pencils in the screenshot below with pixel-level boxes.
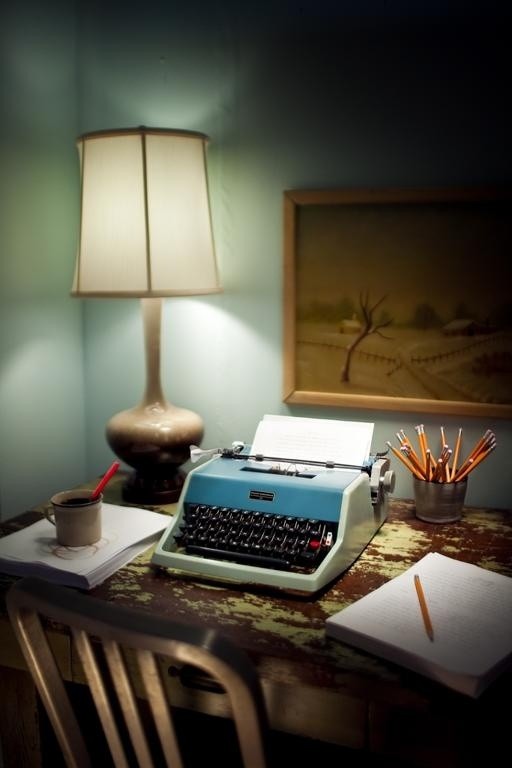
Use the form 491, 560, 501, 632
457, 429, 492, 470
400, 429, 418, 460
484, 439, 495, 449
401, 447, 426, 477
440, 445, 448, 458
440, 427, 451, 483
455, 443, 496, 483
451, 428, 462, 481
452, 433, 495, 480
431, 459, 443, 482
420, 424, 428, 451
415, 575, 434, 643
405, 444, 418, 460
435, 449, 453, 482
430, 453, 436, 466
425, 450, 430, 481
386, 441, 426, 481
395, 433, 405, 447
415, 426, 427, 469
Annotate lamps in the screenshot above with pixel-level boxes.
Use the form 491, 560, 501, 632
69, 126, 223, 506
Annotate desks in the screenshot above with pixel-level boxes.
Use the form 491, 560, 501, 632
0, 469, 512, 768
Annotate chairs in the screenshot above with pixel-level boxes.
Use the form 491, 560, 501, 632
3, 586, 265, 768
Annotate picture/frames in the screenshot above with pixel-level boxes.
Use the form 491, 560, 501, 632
279, 183, 512, 421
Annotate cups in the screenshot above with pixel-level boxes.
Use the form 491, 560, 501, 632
44, 489, 104, 548
412, 468, 468, 524
